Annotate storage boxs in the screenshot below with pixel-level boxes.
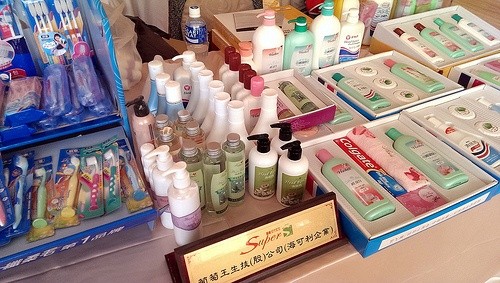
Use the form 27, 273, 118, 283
212, 7, 500, 258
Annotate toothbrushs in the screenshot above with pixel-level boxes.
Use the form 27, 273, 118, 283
0, 0, 114, 138
1, 147, 146, 228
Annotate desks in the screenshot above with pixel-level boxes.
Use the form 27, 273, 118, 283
0, 47, 500, 283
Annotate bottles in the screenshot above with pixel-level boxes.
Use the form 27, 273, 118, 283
427, 116, 500, 169
433, 18, 485, 52
478, 98, 500, 115
314, 148, 396, 221
264, 81, 319, 120
470, 70, 500, 87
384, 128, 468, 189
384, 59, 444, 93
146, 41, 279, 216
332, 73, 390, 110
393, 28, 445, 64
182, 6, 209, 69
451, 13, 500, 47
414, 23, 465, 59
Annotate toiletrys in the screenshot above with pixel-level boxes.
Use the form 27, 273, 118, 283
124, 40, 309, 247
393, 13, 500, 65
330, 59, 446, 111
261, 80, 319, 121
424, 96, 500, 169
314, 125, 469, 222
251, 1, 447, 77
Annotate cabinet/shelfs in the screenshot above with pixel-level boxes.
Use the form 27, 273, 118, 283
1, 0, 158, 272
